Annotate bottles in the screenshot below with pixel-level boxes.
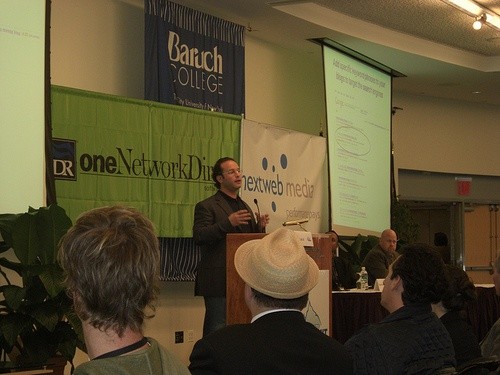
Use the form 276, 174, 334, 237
360, 267, 369, 290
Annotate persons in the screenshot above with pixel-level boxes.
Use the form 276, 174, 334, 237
430, 255, 500, 375
194, 156, 270, 336
324, 232, 352, 295
188, 228, 351, 375
342, 243, 457, 375
53, 203, 191, 375
363, 230, 403, 294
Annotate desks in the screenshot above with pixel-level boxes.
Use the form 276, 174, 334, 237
332, 284, 500, 346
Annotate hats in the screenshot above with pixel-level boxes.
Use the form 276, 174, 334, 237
234, 228, 320, 299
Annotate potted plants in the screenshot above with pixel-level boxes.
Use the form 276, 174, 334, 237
1, 203, 88, 375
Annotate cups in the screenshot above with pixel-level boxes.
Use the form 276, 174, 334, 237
356, 280, 362, 289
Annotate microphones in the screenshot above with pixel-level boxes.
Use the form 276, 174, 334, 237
254, 198, 261, 232
282, 219, 308, 226
